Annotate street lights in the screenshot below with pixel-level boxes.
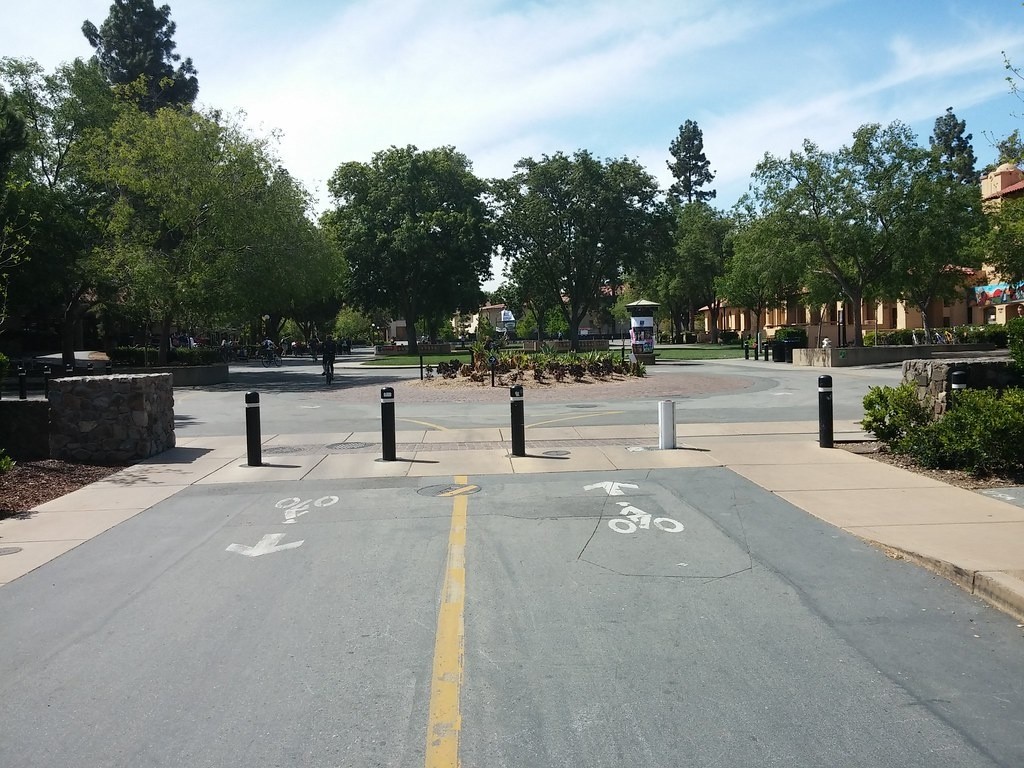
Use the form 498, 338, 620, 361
372, 323, 376, 347
377, 326, 380, 343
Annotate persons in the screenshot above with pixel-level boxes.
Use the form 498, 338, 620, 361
343, 339, 347, 354
310, 335, 320, 361
346, 337, 352, 355
263, 337, 278, 362
337, 338, 343, 355
280, 339, 303, 358
322, 335, 336, 380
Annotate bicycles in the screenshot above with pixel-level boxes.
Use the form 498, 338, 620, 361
261, 348, 282, 367
911, 324, 985, 346
324, 360, 332, 385
310, 348, 318, 364
220, 342, 259, 364
